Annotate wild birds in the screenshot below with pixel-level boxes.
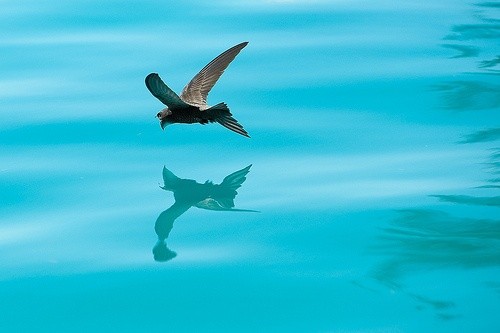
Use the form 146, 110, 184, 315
152, 164, 260, 262
145, 42, 252, 137
